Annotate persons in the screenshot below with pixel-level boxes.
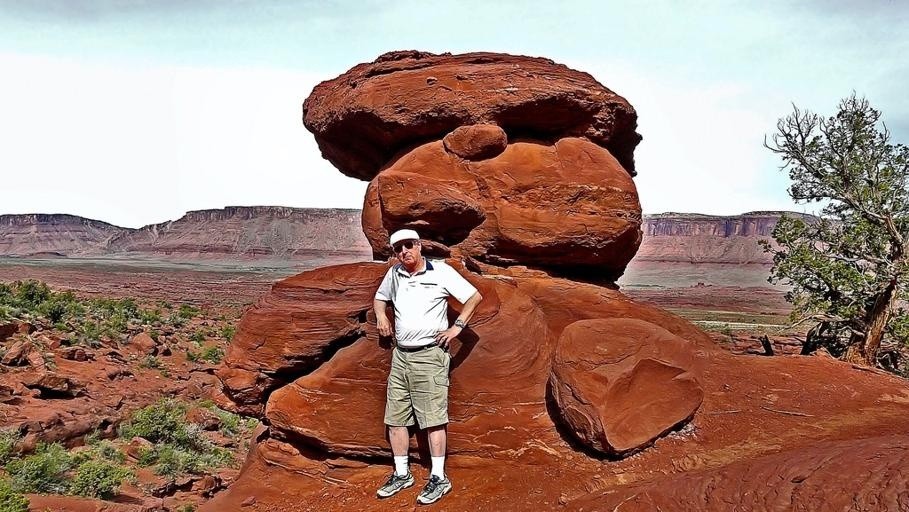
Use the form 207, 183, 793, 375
372, 228, 484, 506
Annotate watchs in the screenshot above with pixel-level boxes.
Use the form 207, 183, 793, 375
455, 319, 465, 329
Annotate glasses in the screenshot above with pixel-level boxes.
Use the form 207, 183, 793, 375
394, 242, 413, 252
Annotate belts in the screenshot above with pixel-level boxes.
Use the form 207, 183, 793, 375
397, 342, 438, 352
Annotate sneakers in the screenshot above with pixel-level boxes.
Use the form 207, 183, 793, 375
417, 474, 452, 504
377, 470, 414, 497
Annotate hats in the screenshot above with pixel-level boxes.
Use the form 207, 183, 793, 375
390, 229, 419, 247
